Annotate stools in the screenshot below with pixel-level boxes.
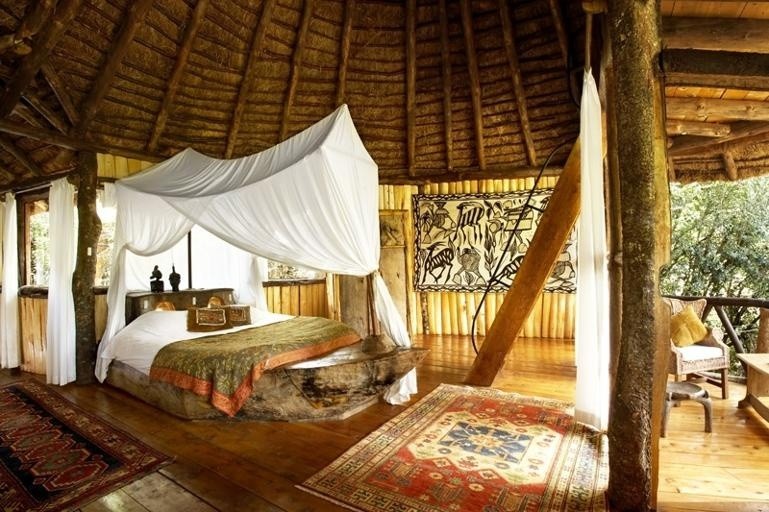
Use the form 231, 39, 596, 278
660, 380, 713, 438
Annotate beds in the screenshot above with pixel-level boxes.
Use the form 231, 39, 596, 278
111, 288, 361, 420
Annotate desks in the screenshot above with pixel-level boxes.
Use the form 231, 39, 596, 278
243, 335, 432, 423
735, 352, 769, 433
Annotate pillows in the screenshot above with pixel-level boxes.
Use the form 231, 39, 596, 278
220, 305, 252, 326
187, 306, 233, 332
670, 303, 707, 348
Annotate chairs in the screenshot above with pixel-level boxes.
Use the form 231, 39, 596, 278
663, 298, 730, 399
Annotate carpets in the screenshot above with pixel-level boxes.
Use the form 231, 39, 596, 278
294, 382, 611, 512
0, 378, 177, 512
